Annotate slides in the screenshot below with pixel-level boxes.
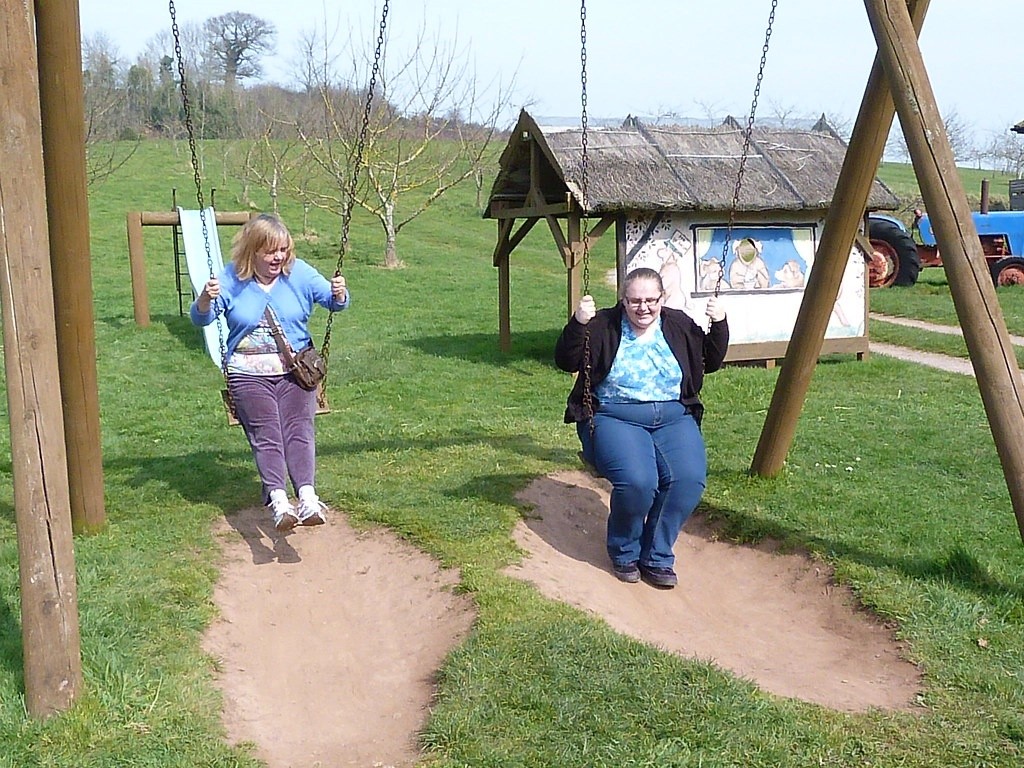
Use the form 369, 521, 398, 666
177, 204, 228, 372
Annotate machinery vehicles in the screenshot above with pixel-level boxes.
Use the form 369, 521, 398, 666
867, 181, 1024, 289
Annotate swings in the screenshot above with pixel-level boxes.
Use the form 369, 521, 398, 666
167, 2, 389, 415
574, 2, 773, 464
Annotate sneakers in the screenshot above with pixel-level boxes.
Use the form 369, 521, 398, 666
614, 563, 641, 582
640, 564, 678, 585
297, 486, 329, 526
268, 489, 299, 531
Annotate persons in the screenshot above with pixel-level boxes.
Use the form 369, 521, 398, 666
189, 213, 350, 531
555, 268, 729, 588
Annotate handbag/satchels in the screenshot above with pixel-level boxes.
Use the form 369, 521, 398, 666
292, 347, 326, 390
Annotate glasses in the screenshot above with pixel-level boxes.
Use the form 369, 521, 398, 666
624, 292, 662, 305
